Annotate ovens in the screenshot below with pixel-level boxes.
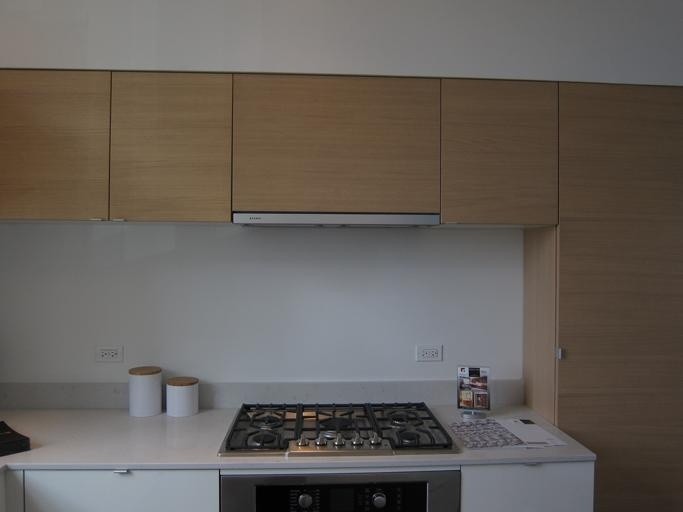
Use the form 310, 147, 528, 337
220, 473, 461, 511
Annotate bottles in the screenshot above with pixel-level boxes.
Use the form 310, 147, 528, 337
128, 366, 162, 416
166, 377, 199, 416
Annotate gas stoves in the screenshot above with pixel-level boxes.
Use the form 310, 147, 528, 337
216, 403, 461, 452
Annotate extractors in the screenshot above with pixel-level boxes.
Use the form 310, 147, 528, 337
232, 213, 440, 225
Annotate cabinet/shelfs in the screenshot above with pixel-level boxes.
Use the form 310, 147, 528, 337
522, 78, 682, 512
458, 462, 594, 512
21, 468, 220, 512
1, 68, 233, 223
231, 71, 557, 232
